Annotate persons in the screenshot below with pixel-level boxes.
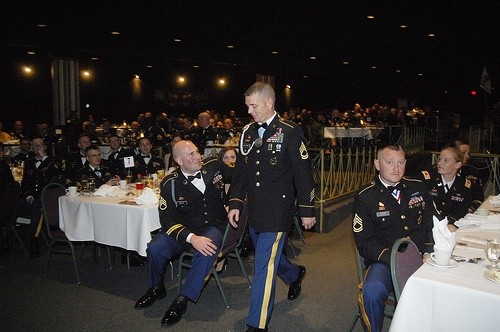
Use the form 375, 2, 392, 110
0, 103, 426, 267
352, 141, 435, 331
420, 139, 484, 233
133, 139, 229, 328
227, 81, 316, 332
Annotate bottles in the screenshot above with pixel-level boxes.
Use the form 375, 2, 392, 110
135, 173, 144, 197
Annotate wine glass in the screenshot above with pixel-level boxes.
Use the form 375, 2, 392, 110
485, 238, 500, 273
126, 170, 132, 186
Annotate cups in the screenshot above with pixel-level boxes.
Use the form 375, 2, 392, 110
206, 141, 213, 144
65, 187, 78, 195
430, 247, 452, 265
474, 208, 490, 216
116, 180, 128, 187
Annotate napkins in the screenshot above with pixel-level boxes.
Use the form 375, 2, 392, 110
94, 183, 122, 197
454, 212, 500, 231
134, 187, 158, 205
431, 214, 457, 250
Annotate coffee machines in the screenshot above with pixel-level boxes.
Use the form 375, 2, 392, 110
89, 179, 96, 197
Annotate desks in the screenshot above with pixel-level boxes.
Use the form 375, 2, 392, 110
323, 126, 383, 139
388, 194, 500, 332
58, 186, 163, 257
98, 146, 131, 160
205, 144, 246, 158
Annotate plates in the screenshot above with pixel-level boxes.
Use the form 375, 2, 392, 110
425, 258, 459, 269
66, 193, 80, 197
484, 268, 500, 283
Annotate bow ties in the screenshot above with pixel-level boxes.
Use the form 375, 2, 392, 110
80, 154, 87, 159
387, 183, 402, 194
187, 172, 202, 184
34, 159, 42, 163
256, 122, 267, 130
23, 152, 30, 156
112, 150, 120, 155
202, 128, 206, 131
141, 155, 150, 160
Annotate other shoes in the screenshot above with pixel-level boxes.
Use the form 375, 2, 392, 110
30, 247, 39, 257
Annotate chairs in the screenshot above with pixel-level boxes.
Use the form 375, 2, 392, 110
349, 237, 398, 332
41, 184, 113, 284
390, 238, 423, 301
178, 203, 252, 309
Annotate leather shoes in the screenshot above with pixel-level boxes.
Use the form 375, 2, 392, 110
245, 325, 268, 332
123, 255, 144, 267
162, 300, 188, 326
287, 266, 306, 300
134, 288, 166, 309
